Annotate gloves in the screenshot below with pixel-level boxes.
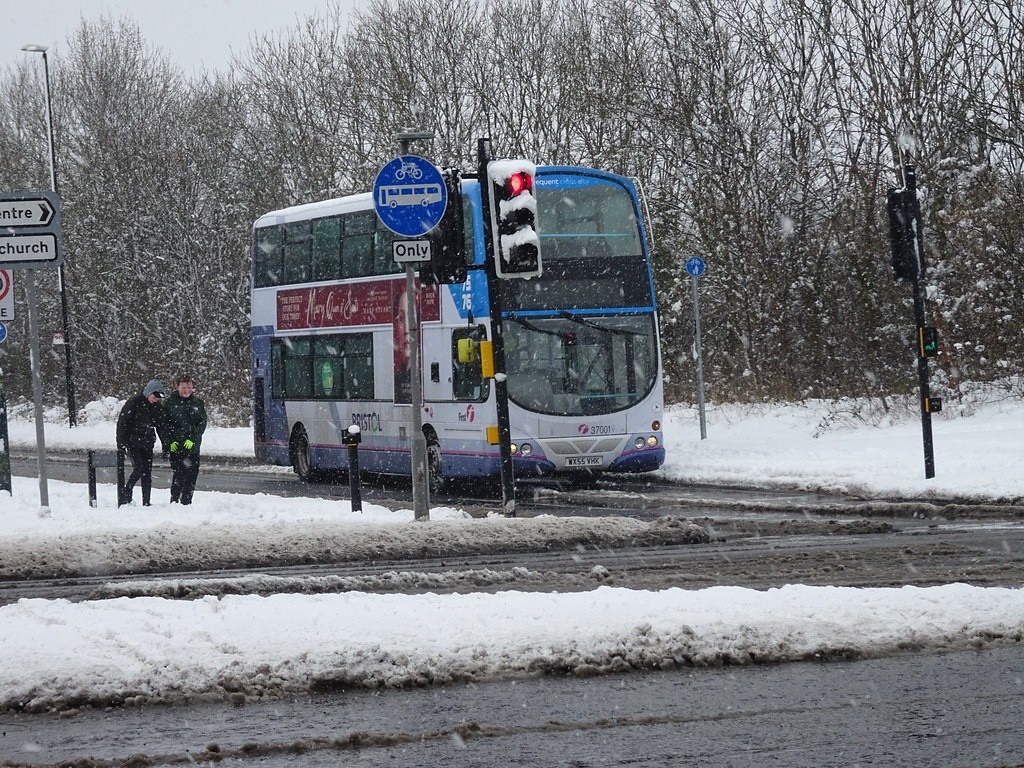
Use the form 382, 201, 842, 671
169, 442, 178, 452
183, 439, 194, 449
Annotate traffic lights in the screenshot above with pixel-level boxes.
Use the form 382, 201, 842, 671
487, 159, 543, 282
925, 397, 943, 412
920, 325, 940, 359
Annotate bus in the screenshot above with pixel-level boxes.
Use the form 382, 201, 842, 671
245, 163, 668, 493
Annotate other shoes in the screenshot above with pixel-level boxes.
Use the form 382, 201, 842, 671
124, 487, 132, 503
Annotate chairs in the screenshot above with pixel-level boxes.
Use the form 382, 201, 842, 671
539, 237, 611, 257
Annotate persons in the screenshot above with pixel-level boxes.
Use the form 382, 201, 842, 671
159, 376, 207, 505
394, 284, 421, 404
117, 380, 169, 506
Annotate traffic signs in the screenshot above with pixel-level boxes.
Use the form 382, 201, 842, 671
0, 198, 57, 229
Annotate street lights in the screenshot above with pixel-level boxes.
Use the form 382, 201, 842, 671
395, 128, 435, 527
20, 43, 76, 428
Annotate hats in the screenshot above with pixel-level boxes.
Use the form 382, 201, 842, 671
154, 391, 165, 398
